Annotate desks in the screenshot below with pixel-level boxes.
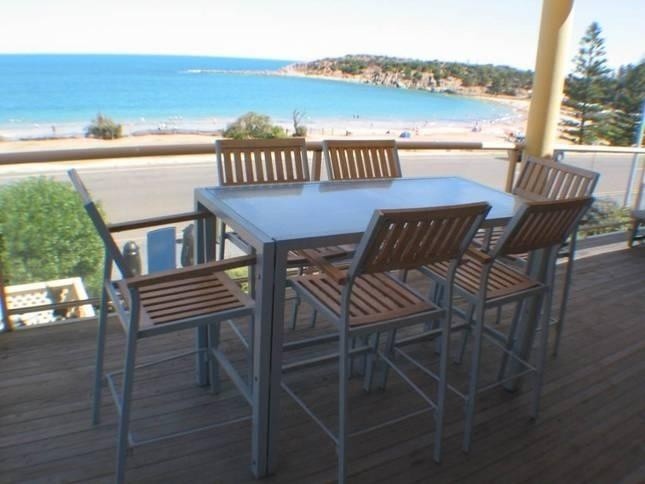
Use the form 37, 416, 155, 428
194, 175, 552, 483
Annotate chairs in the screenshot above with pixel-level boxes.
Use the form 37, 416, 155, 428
379, 194, 598, 454
308, 138, 404, 329
279, 200, 492, 484
629, 210, 645, 248
66, 167, 257, 483
429, 154, 602, 381
214, 136, 346, 352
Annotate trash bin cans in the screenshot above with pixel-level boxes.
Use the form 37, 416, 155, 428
123, 240, 142, 276
